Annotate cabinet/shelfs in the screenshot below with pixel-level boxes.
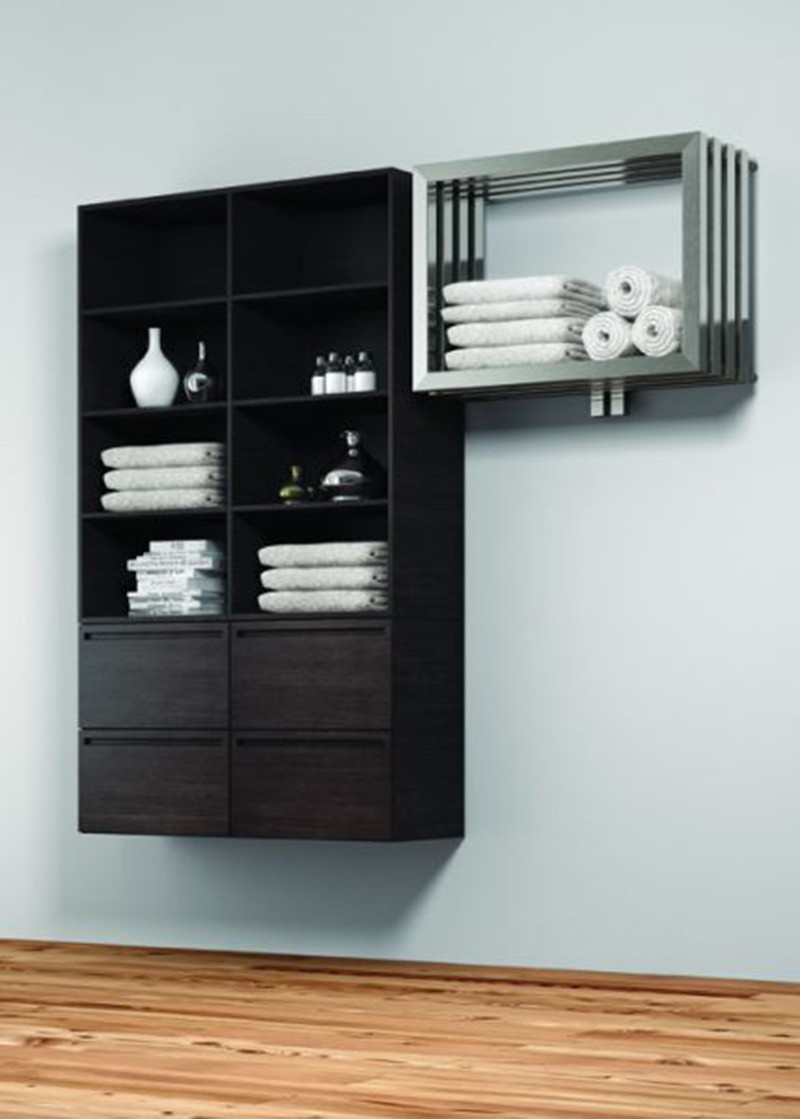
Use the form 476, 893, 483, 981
69, 166, 470, 846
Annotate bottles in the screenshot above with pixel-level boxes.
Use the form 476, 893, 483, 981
312, 351, 377, 395
128, 327, 178, 407
179, 340, 222, 400
318, 428, 389, 500
277, 466, 315, 503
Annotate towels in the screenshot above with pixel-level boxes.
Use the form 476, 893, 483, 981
101, 465, 227, 490
439, 302, 607, 320
629, 304, 687, 357
255, 539, 393, 566
444, 342, 592, 364
603, 264, 687, 314
581, 309, 640, 362
440, 273, 610, 306
100, 442, 226, 466
446, 320, 588, 344
256, 588, 392, 611
258, 561, 391, 593
99, 486, 226, 509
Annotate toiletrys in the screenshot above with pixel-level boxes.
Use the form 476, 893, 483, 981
320, 424, 387, 503
307, 352, 379, 394
276, 444, 323, 503
